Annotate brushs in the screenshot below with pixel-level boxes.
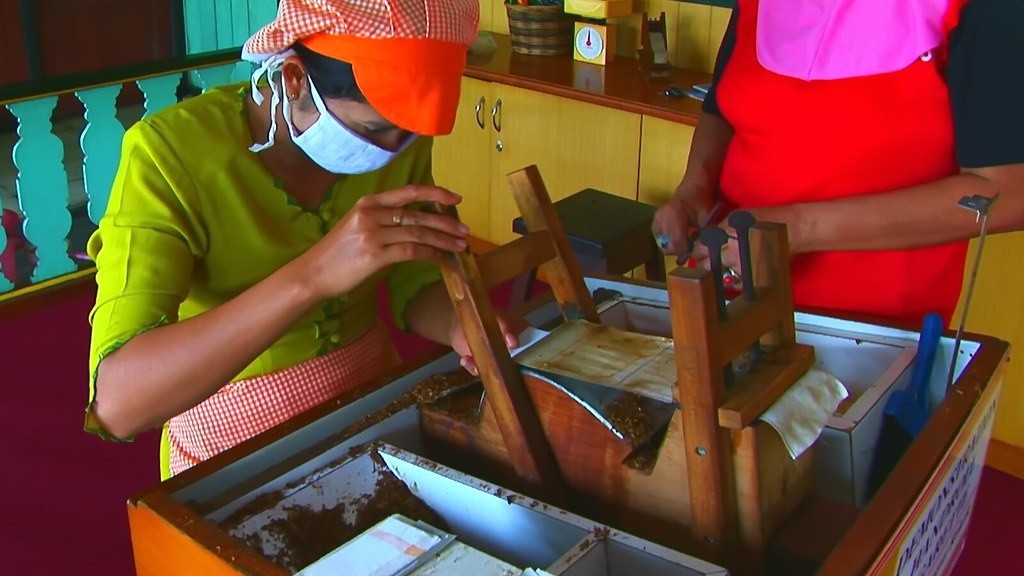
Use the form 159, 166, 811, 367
871, 312, 943, 480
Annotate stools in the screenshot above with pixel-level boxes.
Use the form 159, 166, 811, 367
512, 187, 667, 309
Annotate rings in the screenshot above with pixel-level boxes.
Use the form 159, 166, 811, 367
657, 232, 670, 248
391, 208, 403, 225
724, 267, 740, 283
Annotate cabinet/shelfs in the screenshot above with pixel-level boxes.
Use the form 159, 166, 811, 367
958, 230, 1024, 481
430, 75, 642, 247
641, 111, 983, 340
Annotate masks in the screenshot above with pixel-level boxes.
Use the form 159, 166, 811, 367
293, 110, 420, 175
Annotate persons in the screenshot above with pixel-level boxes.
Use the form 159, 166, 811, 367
84, 0, 521, 486
652, 0, 1024, 331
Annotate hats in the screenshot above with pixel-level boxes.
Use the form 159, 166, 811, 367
241, 0, 480, 136
